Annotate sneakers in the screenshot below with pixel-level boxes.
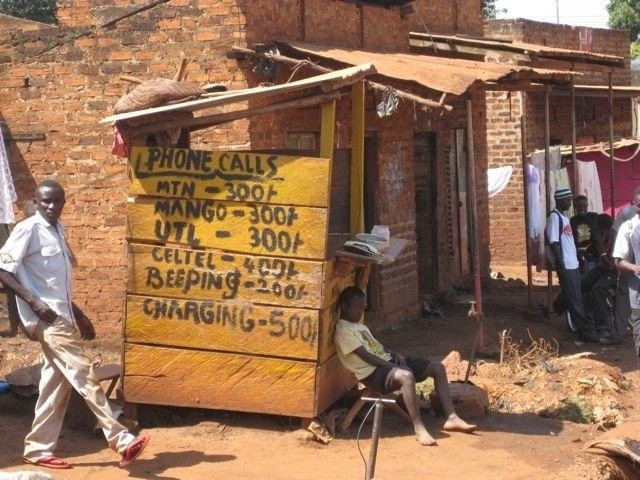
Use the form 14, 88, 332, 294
599, 333, 624, 346
566, 308, 578, 332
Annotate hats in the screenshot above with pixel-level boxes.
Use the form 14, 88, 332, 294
554, 186, 573, 201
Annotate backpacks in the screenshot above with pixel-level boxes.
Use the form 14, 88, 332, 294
543, 210, 563, 271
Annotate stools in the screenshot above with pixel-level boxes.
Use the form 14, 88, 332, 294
339, 383, 411, 432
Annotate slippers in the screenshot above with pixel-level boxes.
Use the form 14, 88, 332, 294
22, 456, 71, 469
118, 434, 153, 468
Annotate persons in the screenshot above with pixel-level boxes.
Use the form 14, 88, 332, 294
534, 186, 640, 365
333, 287, 478, 447
0, 180, 149, 469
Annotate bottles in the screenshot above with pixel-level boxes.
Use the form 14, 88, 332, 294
371, 225, 390, 244
